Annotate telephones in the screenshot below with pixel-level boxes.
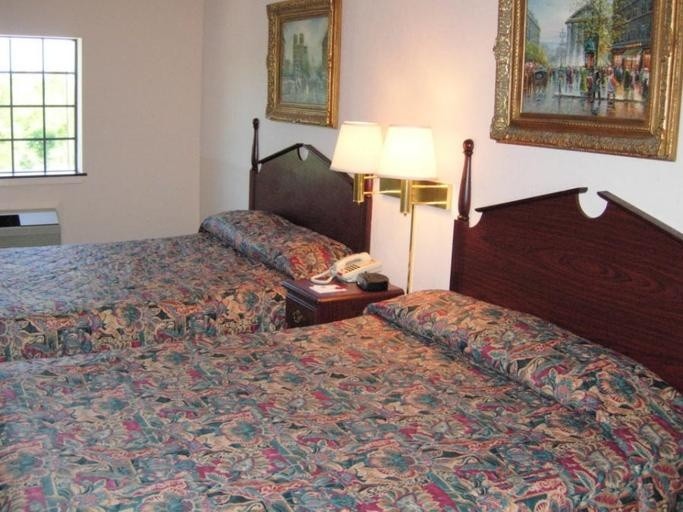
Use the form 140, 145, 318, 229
331, 252, 380, 283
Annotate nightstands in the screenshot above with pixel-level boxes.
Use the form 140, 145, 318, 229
280, 279, 405, 328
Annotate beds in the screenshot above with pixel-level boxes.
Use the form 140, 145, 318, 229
0, 118, 374, 362
0, 139, 683, 512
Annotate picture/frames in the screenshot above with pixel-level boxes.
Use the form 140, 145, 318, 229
490, 0, 683, 161
266, 0, 342, 128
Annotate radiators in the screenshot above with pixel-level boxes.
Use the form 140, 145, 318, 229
0, 210, 61, 247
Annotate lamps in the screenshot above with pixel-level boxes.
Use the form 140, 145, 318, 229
330, 121, 452, 216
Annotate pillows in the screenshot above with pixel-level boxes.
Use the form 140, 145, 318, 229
201, 210, 353, 281
368, 289, 683, 512
